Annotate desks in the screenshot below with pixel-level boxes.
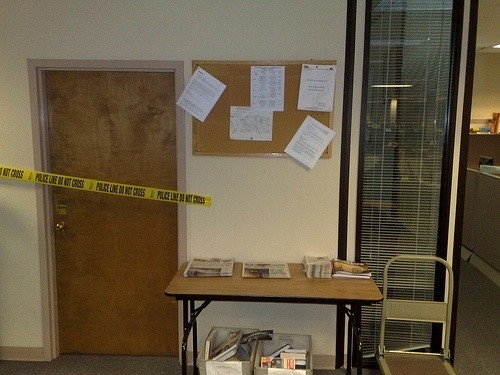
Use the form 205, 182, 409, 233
165, 261, 384, 375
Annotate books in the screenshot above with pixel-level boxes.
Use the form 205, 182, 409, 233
261, 344, 308, 375
303, 255, 372, 279
493, 113, 500, 134
204, 327, 252, 375
183, 256, 234, 277
242, 262, 292, 279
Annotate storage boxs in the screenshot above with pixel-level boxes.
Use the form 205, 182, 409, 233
254, 334, 314, 375
198, 327, 260, 375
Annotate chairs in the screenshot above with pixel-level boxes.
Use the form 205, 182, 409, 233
375, 255, 456, 375
